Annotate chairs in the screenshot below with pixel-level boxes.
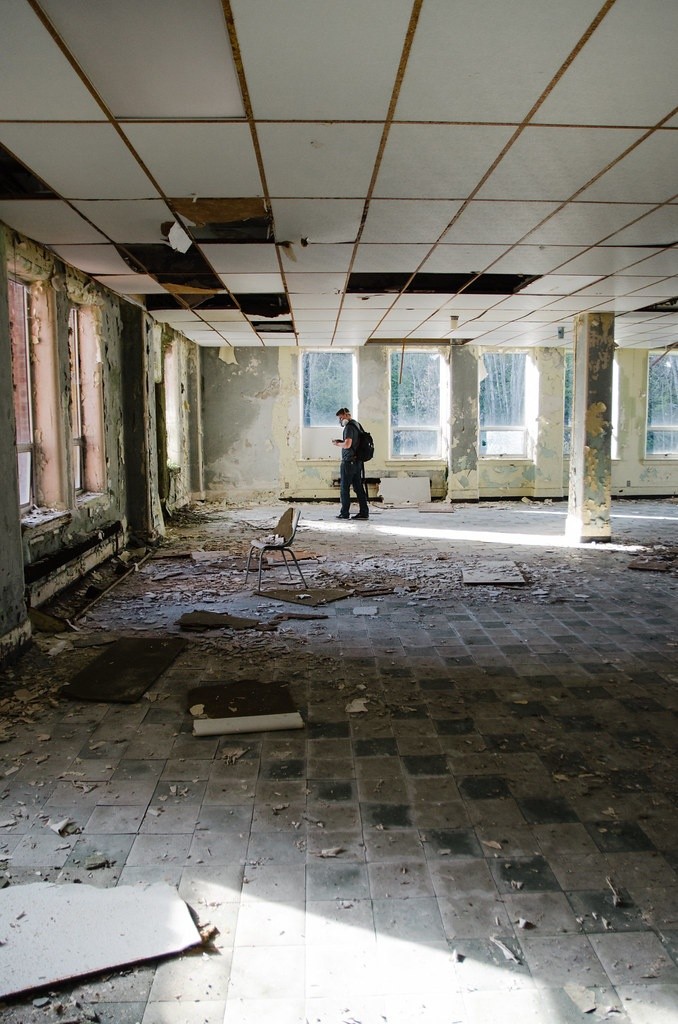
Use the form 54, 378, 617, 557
245, 507, 309, 592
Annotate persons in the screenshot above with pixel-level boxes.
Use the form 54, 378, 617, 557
329, 408, 369, 520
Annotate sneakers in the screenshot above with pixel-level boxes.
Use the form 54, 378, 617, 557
352, 513, 369, 520
336, 513, 351, 520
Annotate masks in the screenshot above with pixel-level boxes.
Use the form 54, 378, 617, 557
340, 418, 349, 427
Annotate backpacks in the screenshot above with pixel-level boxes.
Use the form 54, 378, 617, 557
346, 422, 374, 461
